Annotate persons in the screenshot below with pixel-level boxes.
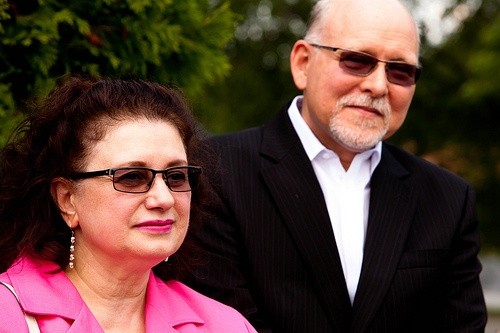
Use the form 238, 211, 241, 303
0, 78, 258, 333
181, 0, 488, 333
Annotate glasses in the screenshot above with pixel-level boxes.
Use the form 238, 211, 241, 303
72, 166, 202, 194
309, 42, 424, 87
